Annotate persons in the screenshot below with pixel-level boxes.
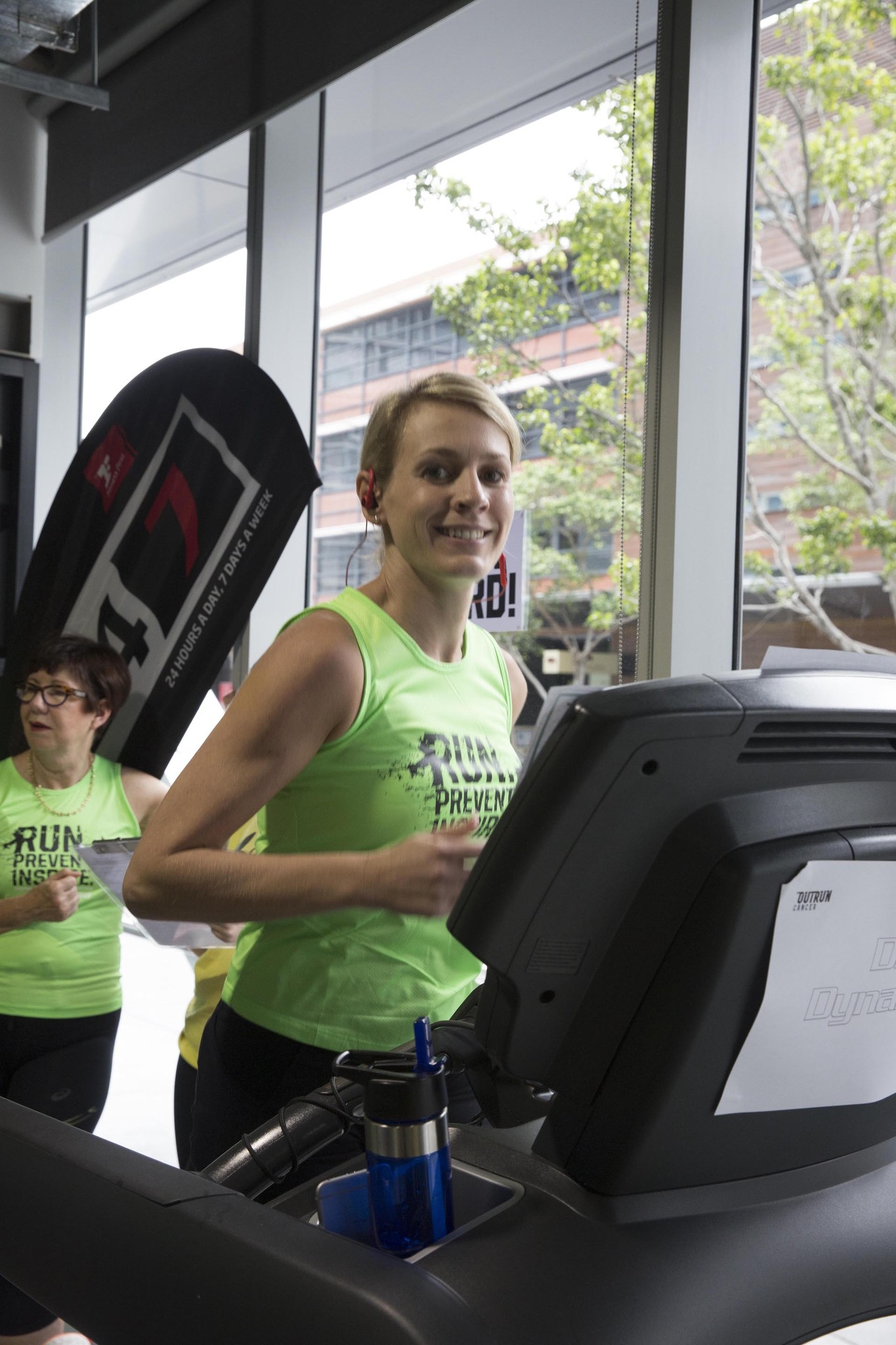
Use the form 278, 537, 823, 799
0, 632, 169, 1345
119, 371, 527, 1170
173, 812, 258, 1171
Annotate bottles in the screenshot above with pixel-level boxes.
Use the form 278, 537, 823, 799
332, 1016, 454, 1252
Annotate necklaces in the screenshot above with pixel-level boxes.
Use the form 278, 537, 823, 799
25, 748, 95, 817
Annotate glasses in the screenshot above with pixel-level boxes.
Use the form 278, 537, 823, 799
16, 681, 89, 707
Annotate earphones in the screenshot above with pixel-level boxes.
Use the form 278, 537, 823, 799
361, 468, 375, 509
